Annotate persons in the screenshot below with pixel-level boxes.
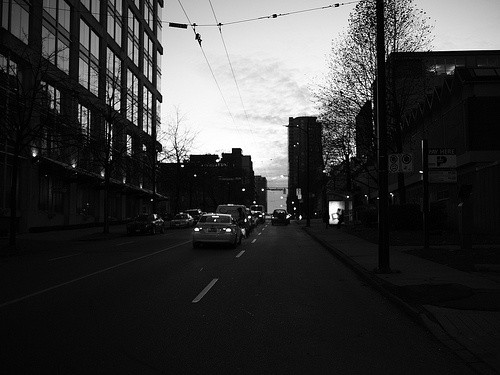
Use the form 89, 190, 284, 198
337, 209, 344, 229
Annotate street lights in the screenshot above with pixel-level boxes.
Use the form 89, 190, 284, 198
285, 125, 310, 227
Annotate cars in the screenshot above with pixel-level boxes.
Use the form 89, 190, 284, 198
192, 213, 243, 249
171, 212, 194, 228
245, 204, 266, 228
127, 213, 166, 235
271, 209, 290, 226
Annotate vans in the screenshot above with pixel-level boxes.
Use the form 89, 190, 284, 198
217, 204, 250, 239
185, 209, 203, 226
